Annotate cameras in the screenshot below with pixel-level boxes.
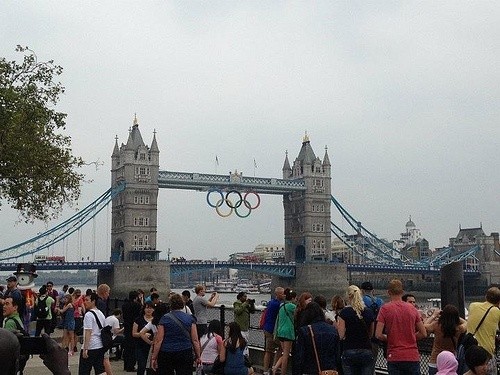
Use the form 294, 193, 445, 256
439, 310, 443, 315
247, 299, 255, 303
213, 292, 217, 296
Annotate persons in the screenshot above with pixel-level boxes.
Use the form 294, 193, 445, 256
0, 277, 500, 375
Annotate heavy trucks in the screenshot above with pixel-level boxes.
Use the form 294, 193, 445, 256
229, 252, 257, 263
34, 254, 66, 265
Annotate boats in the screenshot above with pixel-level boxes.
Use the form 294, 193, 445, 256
426, 298, 469, 317
192, 279, 272, 295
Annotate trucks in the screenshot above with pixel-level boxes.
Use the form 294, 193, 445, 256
171, 257, 186, 263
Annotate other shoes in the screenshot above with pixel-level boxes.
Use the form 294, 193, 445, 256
272, 366, 278, 375
124, 367, 137, 372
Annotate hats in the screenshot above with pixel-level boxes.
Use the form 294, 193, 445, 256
6, 276, 17, 281
361, 282, 373, 290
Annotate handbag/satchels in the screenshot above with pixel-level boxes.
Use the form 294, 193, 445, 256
260, 310, 266, 328
462, 333, 478, 354
320, 370, 339, 375
243, 354, 256, 368
4, 317, 33, 371
90, 310, 115, 352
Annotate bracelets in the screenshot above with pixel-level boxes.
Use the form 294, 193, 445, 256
84, 349, 88, 351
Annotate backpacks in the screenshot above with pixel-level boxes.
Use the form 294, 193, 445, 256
35, 295, 49, 319
365, 294, 387, 345
